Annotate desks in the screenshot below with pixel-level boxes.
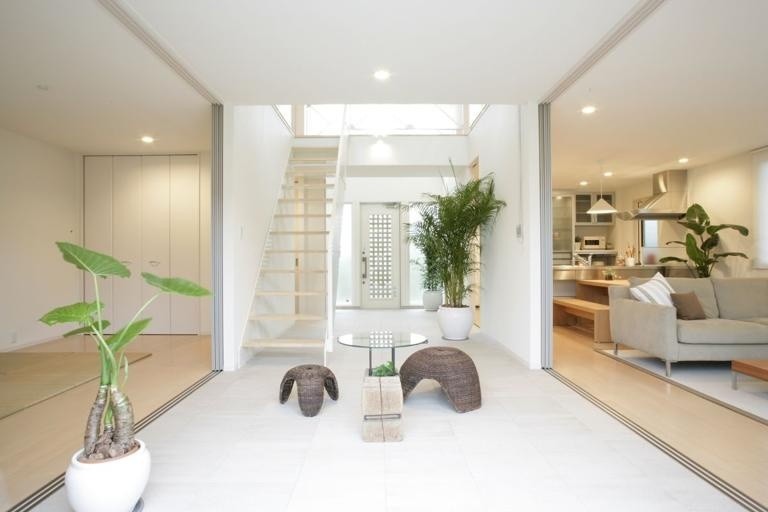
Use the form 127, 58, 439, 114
338, 331, 428, 375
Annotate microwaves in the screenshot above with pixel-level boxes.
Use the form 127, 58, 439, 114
583, 236, 606, 250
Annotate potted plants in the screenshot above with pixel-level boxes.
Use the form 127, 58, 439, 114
38, 241, 212, 512
394, 202, 443, 312
364, 362, 404, 419
575, 236, 582, 250
423, 156, 507, 340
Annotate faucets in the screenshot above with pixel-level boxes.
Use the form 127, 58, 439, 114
570, 252, 593, 267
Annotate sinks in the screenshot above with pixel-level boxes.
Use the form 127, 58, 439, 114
552, 262, 579, 269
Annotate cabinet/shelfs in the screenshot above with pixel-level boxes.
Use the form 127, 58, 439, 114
573, 192, 615, 226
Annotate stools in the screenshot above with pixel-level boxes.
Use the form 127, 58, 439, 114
399, 347, 481, 414
280, 365, 339, 416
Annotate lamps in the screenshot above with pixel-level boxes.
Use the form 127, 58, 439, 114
586, 180, 617, 214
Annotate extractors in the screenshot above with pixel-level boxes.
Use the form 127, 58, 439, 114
616, 169, 688, 220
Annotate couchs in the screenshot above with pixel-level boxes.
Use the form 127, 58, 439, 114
608, 286, 768, 376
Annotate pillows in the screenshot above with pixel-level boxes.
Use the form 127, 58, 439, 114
629, 271, 677, 306
671, 291, 705, 320
711, 278, 768, 318
631, 277, 719, 319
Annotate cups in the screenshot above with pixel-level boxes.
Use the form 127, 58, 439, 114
616, 256, 635, 267
